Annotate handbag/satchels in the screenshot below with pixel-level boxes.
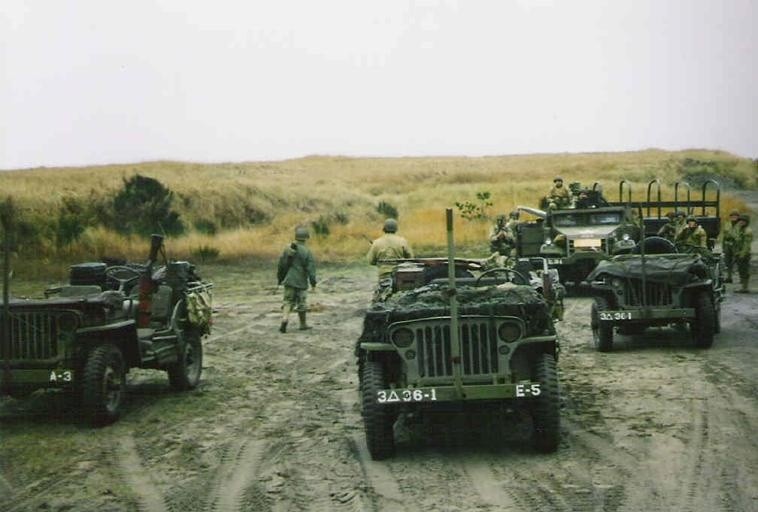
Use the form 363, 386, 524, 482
186, 291, 213, 336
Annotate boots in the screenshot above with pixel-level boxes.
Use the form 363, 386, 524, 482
298, 310, 312, 330
280, 308, 290, 333
734, 278, 749, 293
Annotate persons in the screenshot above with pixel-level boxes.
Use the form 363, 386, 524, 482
368, 218, 414, 283
277, 227, 319, 332
489, 175, 753, 294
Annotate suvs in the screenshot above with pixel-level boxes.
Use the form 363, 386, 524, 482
359, 202, 565, 460
3, 235, 220, 424
589, 232, 727, 350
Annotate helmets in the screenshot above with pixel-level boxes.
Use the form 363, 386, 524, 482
295, 228, 310, 241
677, 211, 685, 216
382, 218, 398, 233
738, 214, 750, 221
686, 215, 696, 224
554, 175, 563, 182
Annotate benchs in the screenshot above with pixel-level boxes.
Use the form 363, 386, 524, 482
430, 275, 508, 288
58, 284, 174, 338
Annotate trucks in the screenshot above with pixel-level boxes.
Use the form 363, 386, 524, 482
513, 181, 724, 290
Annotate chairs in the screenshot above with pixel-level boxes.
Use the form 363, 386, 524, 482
634, 237, 679, 254
